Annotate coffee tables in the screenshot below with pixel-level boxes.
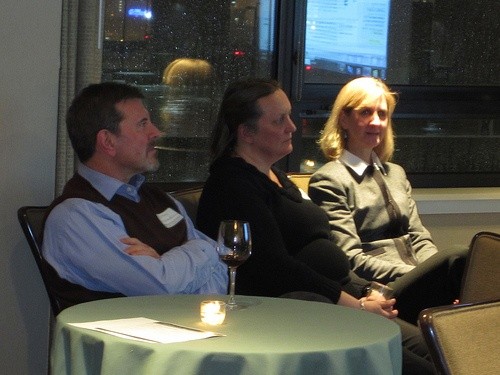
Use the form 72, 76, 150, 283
51, 293, 403, 375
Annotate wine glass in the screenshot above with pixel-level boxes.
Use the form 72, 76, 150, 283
218, 221, 251, 310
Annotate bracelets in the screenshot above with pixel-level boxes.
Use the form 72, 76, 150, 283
359, 300, 365, 311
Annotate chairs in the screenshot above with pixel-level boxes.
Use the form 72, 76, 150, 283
18, 172, 500, 375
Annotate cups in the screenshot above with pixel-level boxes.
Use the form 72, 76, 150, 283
364, 281, 395, 302
197, 266, 226, 328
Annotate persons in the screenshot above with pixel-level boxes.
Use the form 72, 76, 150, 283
196, 77, 470, 375
308, 76, 439, 282
155, 52, 224, 185
40, 81, 229, 307
